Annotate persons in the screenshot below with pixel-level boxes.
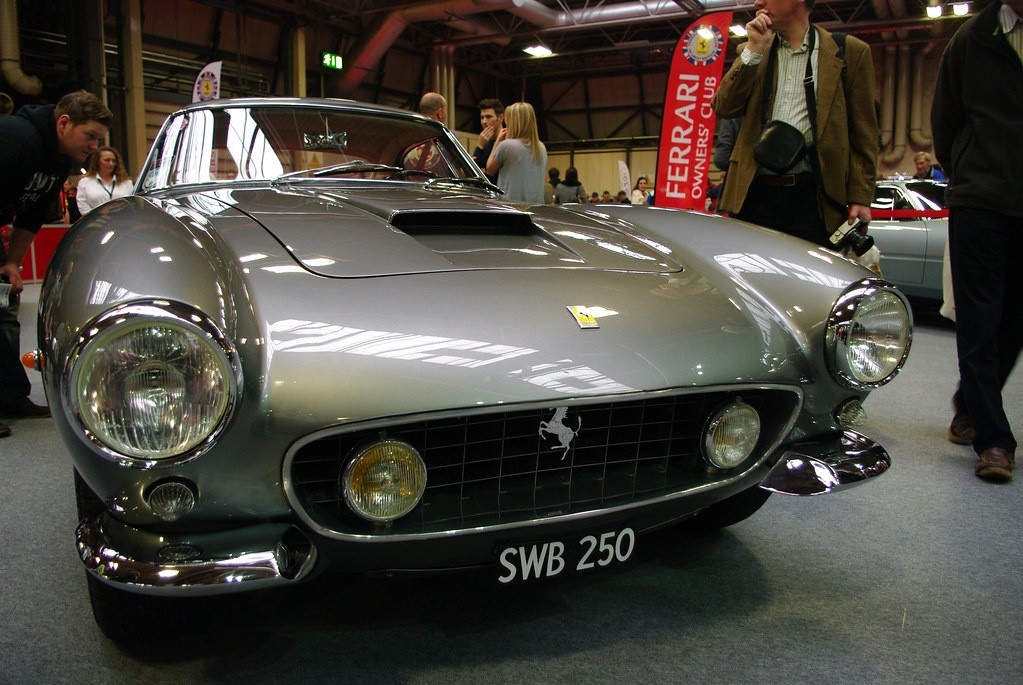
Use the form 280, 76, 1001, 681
913, 0, 1023, 478
705, 0, 882, 253
56, 146, 135, 225
404, 92, 656, 207
0, 91, 111, 438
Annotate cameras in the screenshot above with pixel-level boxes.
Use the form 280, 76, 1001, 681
830, 217, 874, 258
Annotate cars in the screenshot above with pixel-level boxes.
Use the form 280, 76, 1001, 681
846, 176, 950, 303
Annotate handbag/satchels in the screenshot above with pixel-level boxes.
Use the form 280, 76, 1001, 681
752, 120, 806, 173
576, 184, 589, 203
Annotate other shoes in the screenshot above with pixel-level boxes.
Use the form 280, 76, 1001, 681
0, 397, 50, 434
949, 414, 1016, 478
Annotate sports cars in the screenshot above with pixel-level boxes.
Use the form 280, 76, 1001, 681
21, 94, 919, 643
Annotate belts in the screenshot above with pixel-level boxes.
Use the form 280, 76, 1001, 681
752, 171, 815, 186
